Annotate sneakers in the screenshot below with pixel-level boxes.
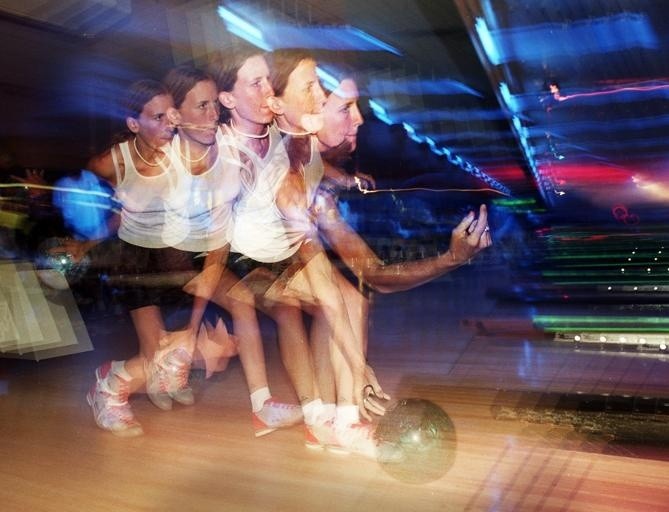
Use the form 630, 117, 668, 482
86, 360, 142, 438
254, 399, 403, 462
145, 347, 194, 411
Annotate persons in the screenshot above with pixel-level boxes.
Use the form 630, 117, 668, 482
10, 47, 492, 457
